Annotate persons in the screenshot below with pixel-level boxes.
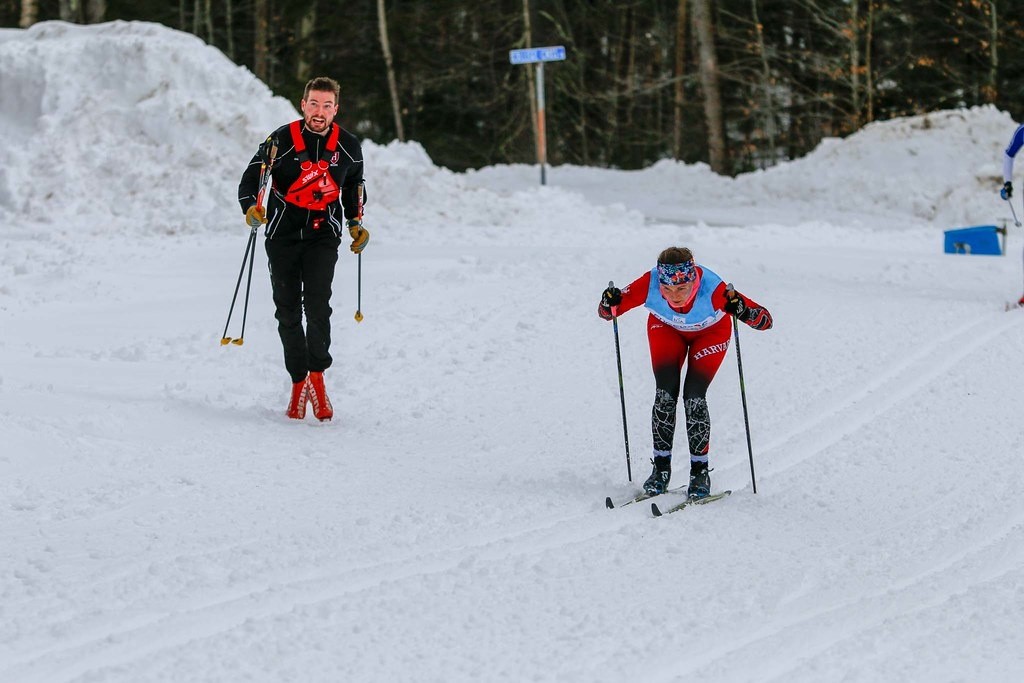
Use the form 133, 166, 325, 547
1000, 123, 1024, 307
239, 77, 368, 419
599, 245, 773, 498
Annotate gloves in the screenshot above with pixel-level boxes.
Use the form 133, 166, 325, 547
245, 205, 268, 226
723, 291, 750, 322
349, 217, 369, 253
602, 287, 623, 310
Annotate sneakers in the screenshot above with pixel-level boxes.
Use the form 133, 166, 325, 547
307, 370, 334, 419
287, 376, 309, 419
643, 457, 672, 494
689, 467, 714, 499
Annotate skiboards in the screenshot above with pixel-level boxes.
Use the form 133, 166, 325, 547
605, 467, 731, 517
1004, 301, 1022, 312
